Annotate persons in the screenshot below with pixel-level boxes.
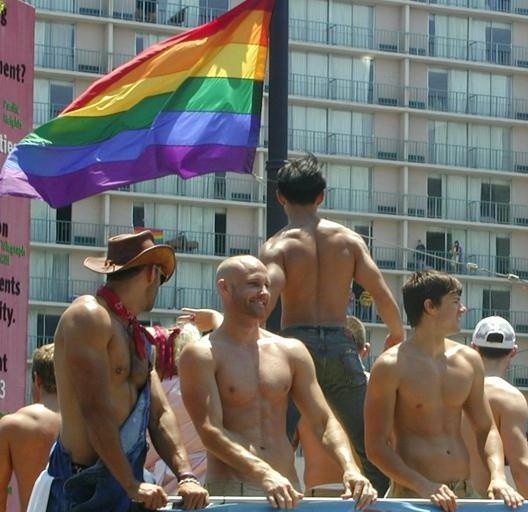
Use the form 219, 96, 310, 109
449, 240, 463, 273
460, 315, 528, 499
176, 151, 406, 498
364, 268, 524, 512
44, 228, 211, 512
177, 254, 380, 512
291, 316, 372, 497
0, 342, 60, 512
413, 239, 427, 269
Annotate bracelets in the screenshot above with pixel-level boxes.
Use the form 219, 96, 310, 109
178, 472, 199, 480
178, 477, 202, 487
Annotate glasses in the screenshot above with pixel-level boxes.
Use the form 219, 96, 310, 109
157, 266, 168, 285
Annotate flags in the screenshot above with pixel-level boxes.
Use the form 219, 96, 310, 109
0, 0, 275, 210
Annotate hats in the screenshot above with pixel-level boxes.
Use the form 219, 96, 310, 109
472, 316, 516, 350
83, 229, 176, 283
